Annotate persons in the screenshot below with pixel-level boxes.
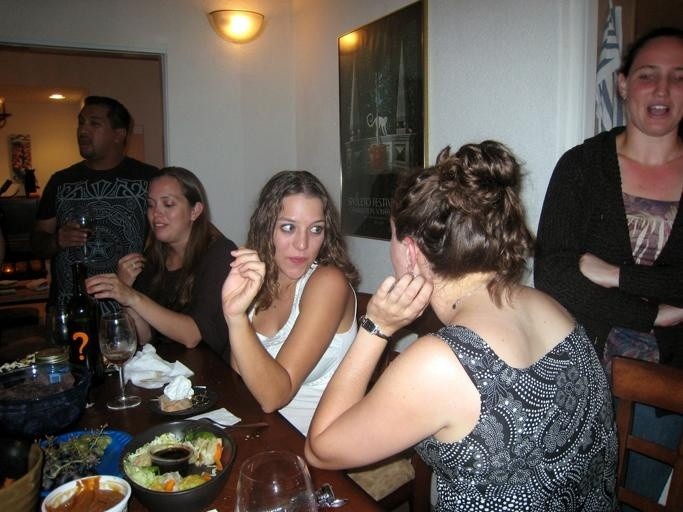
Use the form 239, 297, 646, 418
85, 166, 239, 367
534, 25, 683, 512
30, 96, 160, 343
304, 140, 620, 512
222, 171, 361, 439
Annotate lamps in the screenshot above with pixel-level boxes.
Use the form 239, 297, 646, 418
202, 6, 267, 46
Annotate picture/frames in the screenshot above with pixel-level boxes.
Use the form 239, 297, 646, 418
332, 1, 433, 245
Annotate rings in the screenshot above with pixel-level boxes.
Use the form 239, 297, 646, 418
131, 263, 137, 270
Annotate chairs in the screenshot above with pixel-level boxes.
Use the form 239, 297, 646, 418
340, 285, 440, 511
596, 348, 683, 511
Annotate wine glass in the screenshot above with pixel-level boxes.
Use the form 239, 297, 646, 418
70, 203, 98, 264
98, 309, 142, 409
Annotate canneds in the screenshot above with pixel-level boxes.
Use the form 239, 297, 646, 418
35, 347, 72, 385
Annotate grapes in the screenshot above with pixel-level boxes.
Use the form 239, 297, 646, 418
41, 434, 112, 489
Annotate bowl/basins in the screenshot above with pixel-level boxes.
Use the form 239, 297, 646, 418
41, 474, 132, 511
120, 420, 237, 511
0, 364, 90, 439
0, 443, 42, 511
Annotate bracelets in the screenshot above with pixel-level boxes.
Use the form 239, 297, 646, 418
358, 315, 392, 342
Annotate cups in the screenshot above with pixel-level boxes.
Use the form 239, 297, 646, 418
147, 443, 193, 475
48, 304, 69, 354
237, 450, 316, 511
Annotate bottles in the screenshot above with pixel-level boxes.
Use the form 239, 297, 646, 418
67, 259, 105, 383
24, 168, 35, 196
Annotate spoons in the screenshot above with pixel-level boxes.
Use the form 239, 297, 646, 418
197, 417, 269, 428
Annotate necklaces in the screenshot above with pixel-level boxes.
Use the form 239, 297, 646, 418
451, 282, 487, 310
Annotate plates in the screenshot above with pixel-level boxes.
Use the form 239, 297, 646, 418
37, 428, 134, 495
150, 386, 219, 418
